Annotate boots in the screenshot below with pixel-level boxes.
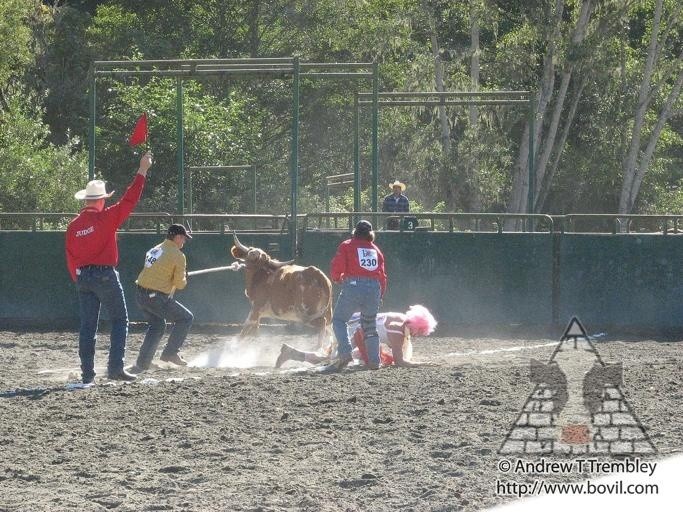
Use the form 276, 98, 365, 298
276, 343, 306, 368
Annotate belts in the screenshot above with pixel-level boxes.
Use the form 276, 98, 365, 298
80, 265, 110, 269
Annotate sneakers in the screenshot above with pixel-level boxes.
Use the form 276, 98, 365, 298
361, 360, 379, 371
336, 353, 353, 370
82, 376, 94, 384
108, 368, 137, 380
140, 361, 163, 369
160, 353, 188, 366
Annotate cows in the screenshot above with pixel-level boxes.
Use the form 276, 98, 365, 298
230, 230, 330, 352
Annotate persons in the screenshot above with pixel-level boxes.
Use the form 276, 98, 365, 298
135, 222, 194, 372
65, 149, 153, 385
382, 181, 410, 230
274, 305, 438, 367
329, 219, 386, 370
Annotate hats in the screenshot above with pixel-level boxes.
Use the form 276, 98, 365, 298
389, 181, 406, 191
73, 179, 115, 200
167, 224, 193, 239
